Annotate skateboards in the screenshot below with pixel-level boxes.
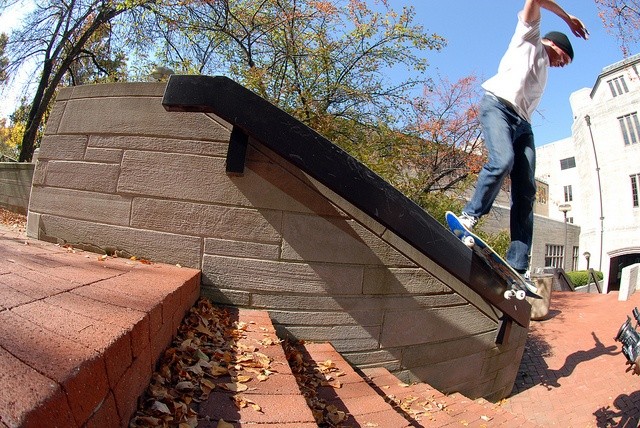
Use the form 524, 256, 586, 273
445, 211, 544, 301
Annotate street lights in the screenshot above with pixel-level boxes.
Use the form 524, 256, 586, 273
582, 252, 591, 270
584, 114, 604, 272
559, 204, 572, 272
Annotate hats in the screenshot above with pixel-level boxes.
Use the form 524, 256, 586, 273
543, 32, 574, 62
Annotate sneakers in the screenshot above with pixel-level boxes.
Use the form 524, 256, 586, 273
518, 269, 538, 292
458, 211, 477, 229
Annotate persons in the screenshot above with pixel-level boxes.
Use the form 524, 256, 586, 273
459, 1, 589, 277
